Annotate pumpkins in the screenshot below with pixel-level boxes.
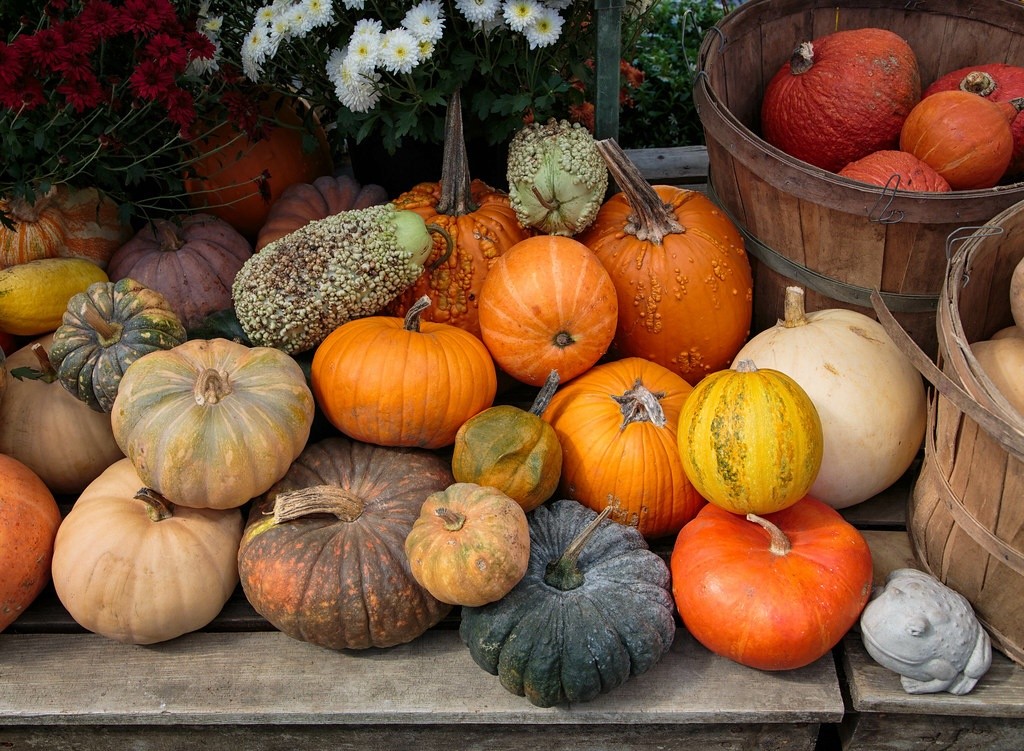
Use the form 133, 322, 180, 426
1, 27, 1024, 707
